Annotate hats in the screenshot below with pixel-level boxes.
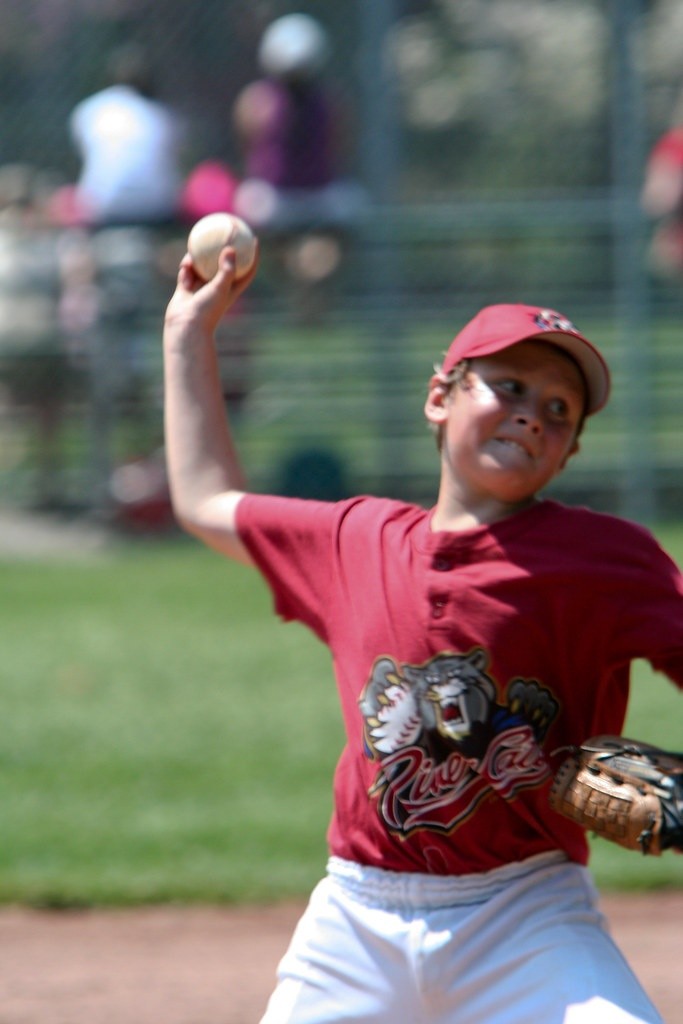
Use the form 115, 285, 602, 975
441, 304, 611, 417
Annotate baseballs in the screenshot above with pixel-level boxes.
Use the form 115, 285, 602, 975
187, 208, 257, 284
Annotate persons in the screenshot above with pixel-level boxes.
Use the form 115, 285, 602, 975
68, 48, 203, 530
640, 129, 682, 280
221, 13, 404, 250
161, 247, 683, 1024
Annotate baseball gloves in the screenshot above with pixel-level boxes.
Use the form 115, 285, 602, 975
550, 735, 683, 856
